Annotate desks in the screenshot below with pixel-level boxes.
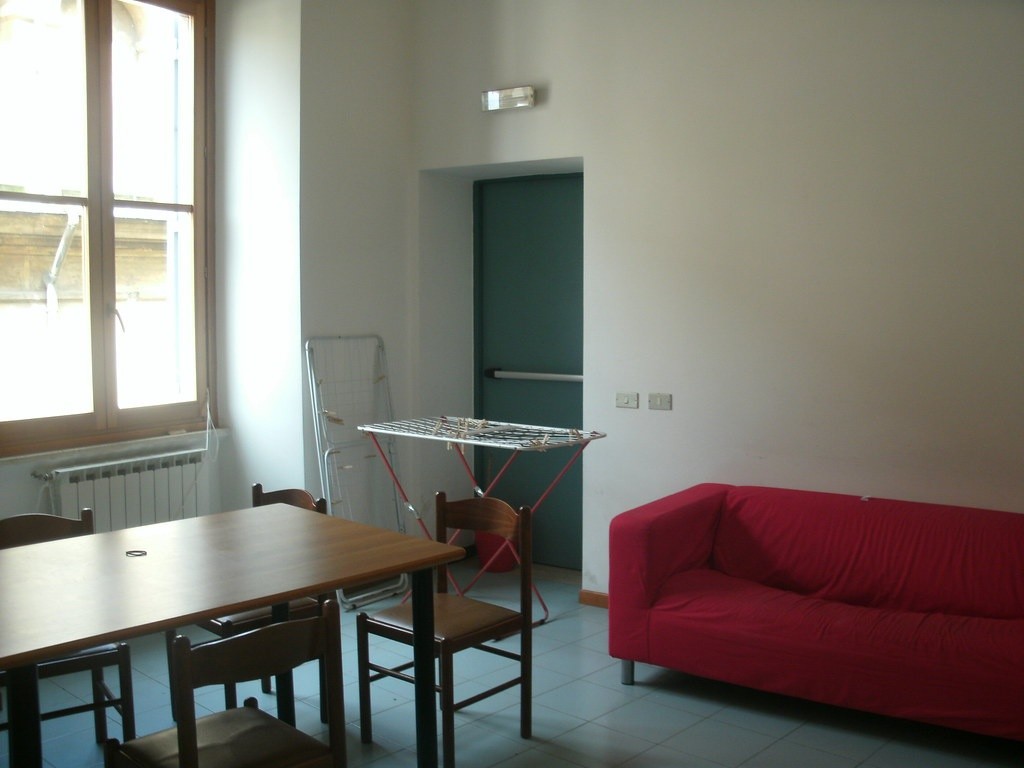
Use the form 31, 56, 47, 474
0, 502, 464, 768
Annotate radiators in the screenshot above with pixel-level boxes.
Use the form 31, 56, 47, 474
49, 449, 207, 530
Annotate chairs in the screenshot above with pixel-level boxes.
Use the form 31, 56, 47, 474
163, 482, 326, 710
356, 491, 532, 768
92, 598, 346, 768
0, 506, 134, 768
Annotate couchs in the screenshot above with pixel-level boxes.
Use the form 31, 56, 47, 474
610, 480, 1023, 742
